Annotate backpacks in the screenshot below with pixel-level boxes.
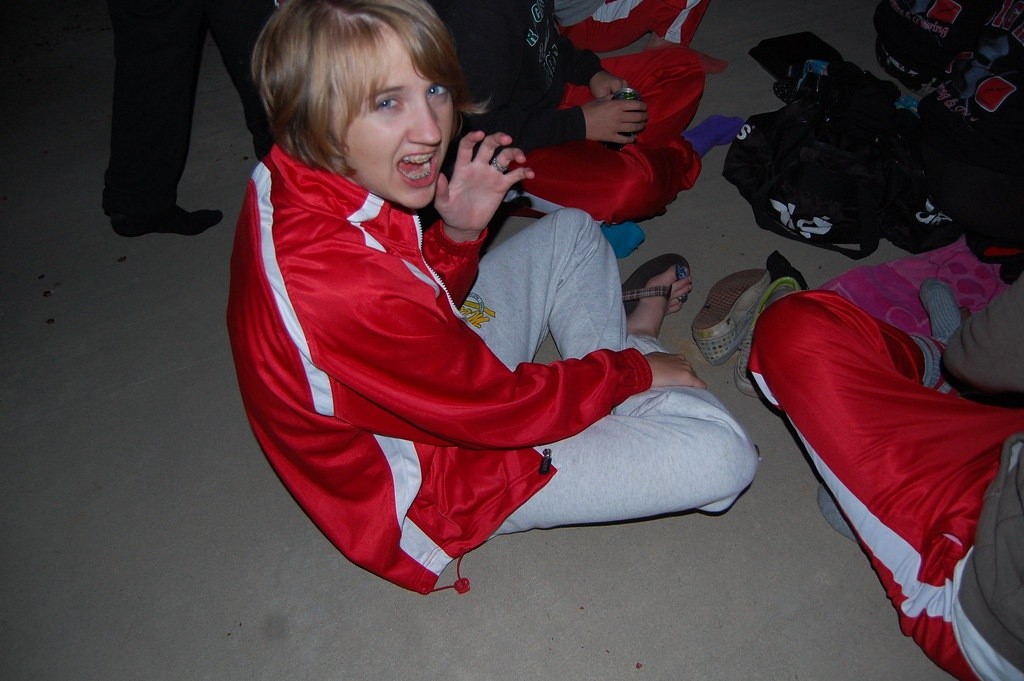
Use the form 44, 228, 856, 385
873, 0, 1024, 285
723, 101, 879, 260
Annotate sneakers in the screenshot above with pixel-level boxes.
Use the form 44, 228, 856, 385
736, 277, 801, 398
690, 269, 770, 365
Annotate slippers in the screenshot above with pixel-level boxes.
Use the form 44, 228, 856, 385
622, 252, 691, 318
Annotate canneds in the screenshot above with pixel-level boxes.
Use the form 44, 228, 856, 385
607, 88, 641, 150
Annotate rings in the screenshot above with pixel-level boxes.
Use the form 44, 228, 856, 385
492, 157, 508, 171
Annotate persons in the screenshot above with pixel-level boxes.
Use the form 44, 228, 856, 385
103, 0, 281, 236
428, 0, 745, 225
227, 0, 758, 595
747, 275, 1024, 680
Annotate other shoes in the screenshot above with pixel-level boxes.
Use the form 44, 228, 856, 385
774, 62, 901, 106
